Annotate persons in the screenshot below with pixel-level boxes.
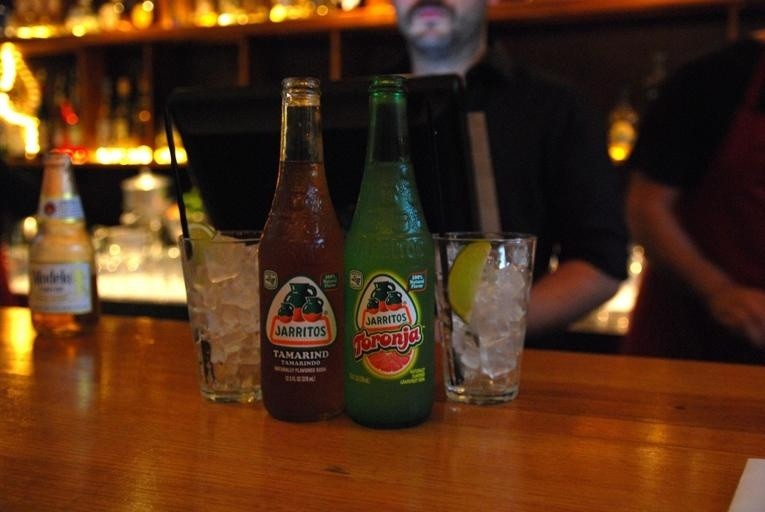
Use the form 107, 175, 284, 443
333, 0, 628, 356
616, 0, 764, 373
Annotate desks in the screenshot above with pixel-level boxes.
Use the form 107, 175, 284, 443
0, 306, 764, 507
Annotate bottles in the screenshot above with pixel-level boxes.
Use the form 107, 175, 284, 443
26, 154, 99, 338
257, 73, 344, 423
341, 75, 436, 429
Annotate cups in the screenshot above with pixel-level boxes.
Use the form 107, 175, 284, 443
433, 230, 536, 403
178, 232, 264, 403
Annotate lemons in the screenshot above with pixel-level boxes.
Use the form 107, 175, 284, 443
444, 241, 494, 324
188, 222, 219, 263
